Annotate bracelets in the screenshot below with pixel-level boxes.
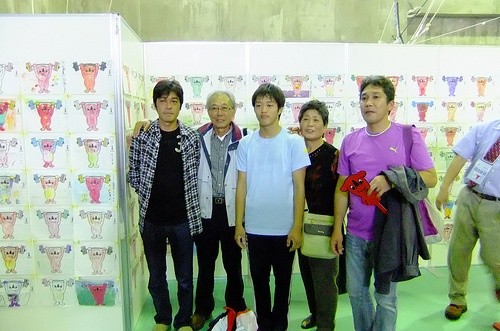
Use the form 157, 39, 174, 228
148, 119, 154, 124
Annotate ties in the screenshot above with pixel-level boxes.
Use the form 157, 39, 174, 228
469, 137, 500, 189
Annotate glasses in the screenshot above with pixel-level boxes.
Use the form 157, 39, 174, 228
208, 105, 235, 112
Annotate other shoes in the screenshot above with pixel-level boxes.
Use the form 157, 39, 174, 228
493, 322, 500, 330
301, 313, 316, 329
496, 289, 500, 302
151, 324, 170, 331
178, 326, 193, 331
191, 313, 212, 331
445, 304, 467, 320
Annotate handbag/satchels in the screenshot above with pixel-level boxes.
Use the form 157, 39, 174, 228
300, 212, 337, 259
419, 197, 444, 244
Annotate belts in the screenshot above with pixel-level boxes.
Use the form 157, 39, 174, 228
212, 197, 225, 204
466, 185, 500, 202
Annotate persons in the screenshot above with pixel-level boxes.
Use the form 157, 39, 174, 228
132, 91, 299, 331
126, 78, 205, 331
331, 76, 438, 331
232, 82, 312, 331
435, 114, 500, 330
297, 100, 351, 331
